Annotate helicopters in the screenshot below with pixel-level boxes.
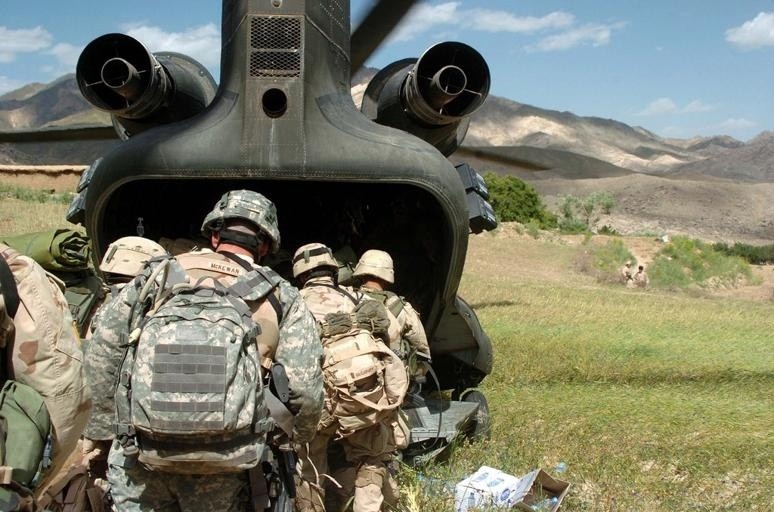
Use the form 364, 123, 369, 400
0, 0, 503, 454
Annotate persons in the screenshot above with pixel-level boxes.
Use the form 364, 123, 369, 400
632, 265, 648, 289
618, 260, 632, 285
1, 189, 432, 512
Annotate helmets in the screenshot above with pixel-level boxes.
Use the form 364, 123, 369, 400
291, 242, 339, 278
99, 236, 169, 278
200, 188, 281, 254
352, 248, 396, 284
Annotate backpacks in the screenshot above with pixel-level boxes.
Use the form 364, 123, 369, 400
300, 287, 411, 440
114, 255, 286, 475
0, 378, 50, 512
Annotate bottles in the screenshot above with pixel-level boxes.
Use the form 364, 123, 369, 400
555, 460, 567, 473
531, 496, 558, 511
